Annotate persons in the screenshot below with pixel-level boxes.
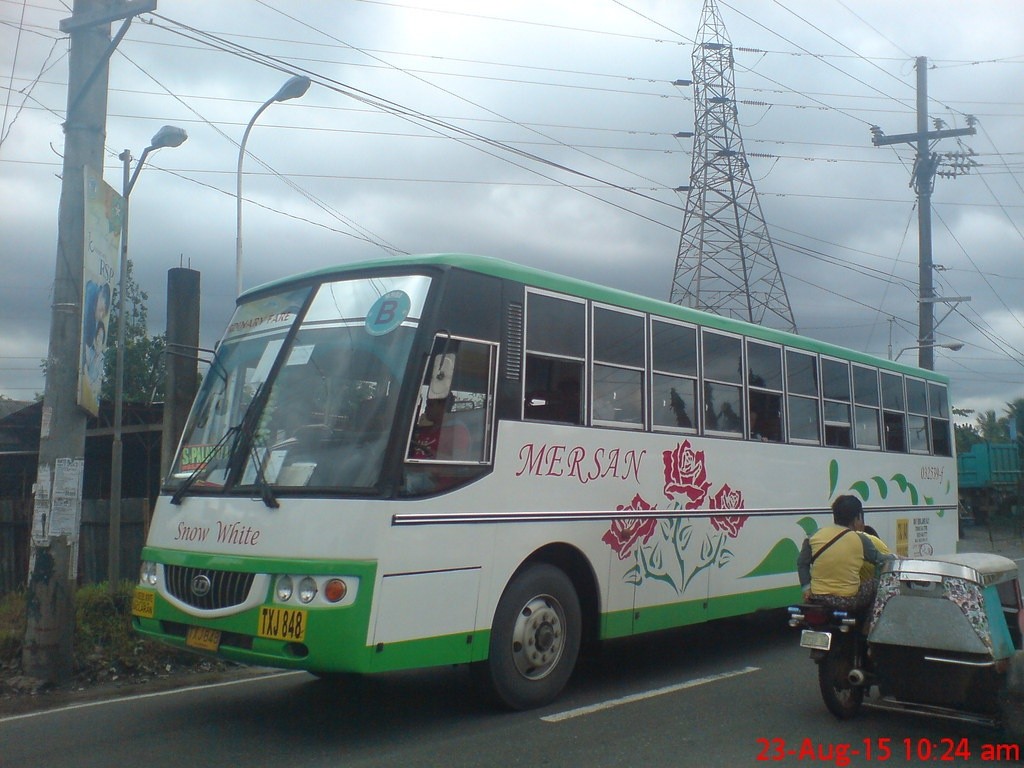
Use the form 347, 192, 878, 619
410, 391, 468, 488
797, 495, 906, 635
748, 411, 769, 442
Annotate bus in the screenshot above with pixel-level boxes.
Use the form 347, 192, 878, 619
130, 254, 960, 713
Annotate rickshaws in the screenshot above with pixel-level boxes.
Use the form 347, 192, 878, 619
786, 552, 1023, 747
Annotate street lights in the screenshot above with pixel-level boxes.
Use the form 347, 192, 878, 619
236, 77, 312, 302
105, 122, 188, 600
